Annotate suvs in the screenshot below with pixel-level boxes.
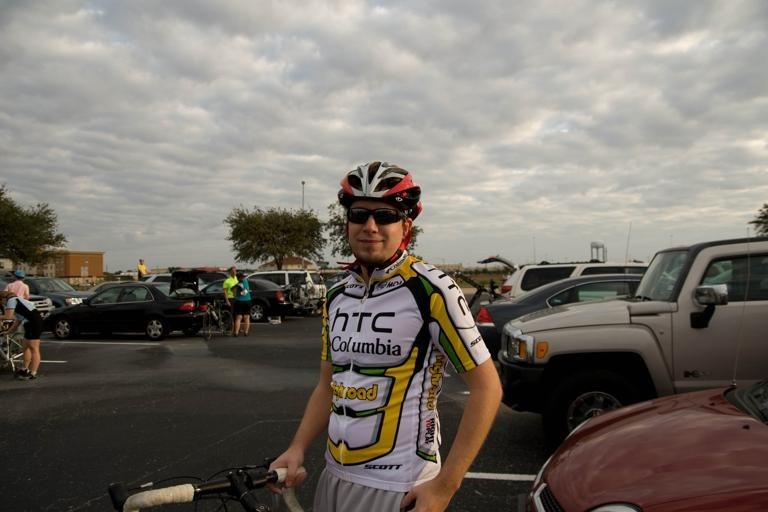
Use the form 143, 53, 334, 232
0, 270, 55, 327
496, 235, 767, 445
501, 258, 734, 302
23, 275, 97, 307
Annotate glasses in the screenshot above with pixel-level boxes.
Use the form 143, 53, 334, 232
347, 208, 400, 224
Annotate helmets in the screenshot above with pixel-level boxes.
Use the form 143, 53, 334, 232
14, 269, 25, 277
338, 162, 422, 220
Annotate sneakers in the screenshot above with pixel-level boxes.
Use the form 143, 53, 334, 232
24, 373, 37, 379
17, 369, 27, 376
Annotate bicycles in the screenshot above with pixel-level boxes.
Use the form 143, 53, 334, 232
449, 270, 505, 310
283, 275, 317, 311
107, 457, 310, 512
202, 298, 234, 342
0, 319, 24, 371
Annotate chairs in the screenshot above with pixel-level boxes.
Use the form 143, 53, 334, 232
125, 293, 136, 301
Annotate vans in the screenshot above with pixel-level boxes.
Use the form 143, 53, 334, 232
246, 269, 327, 311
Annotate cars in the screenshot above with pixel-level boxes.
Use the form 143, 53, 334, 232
88, 280, 148, 302
201, 278, 295, 324
201, 272, 229, 283
43, 281, 209, 341
324, 275, 342, 289
137, 274, 206, 298
526, 370, 767, 511
473, 273, 678, 361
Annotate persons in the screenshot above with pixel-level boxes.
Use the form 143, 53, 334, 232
233, 272, 252, 337
265, 159, 503, 512
223, 266, 239, 332
4, 269, 30, 300
137, 258, 151, 280
0, 289, 44, 380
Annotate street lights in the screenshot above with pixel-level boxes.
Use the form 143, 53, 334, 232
300, 180, 307, 268
